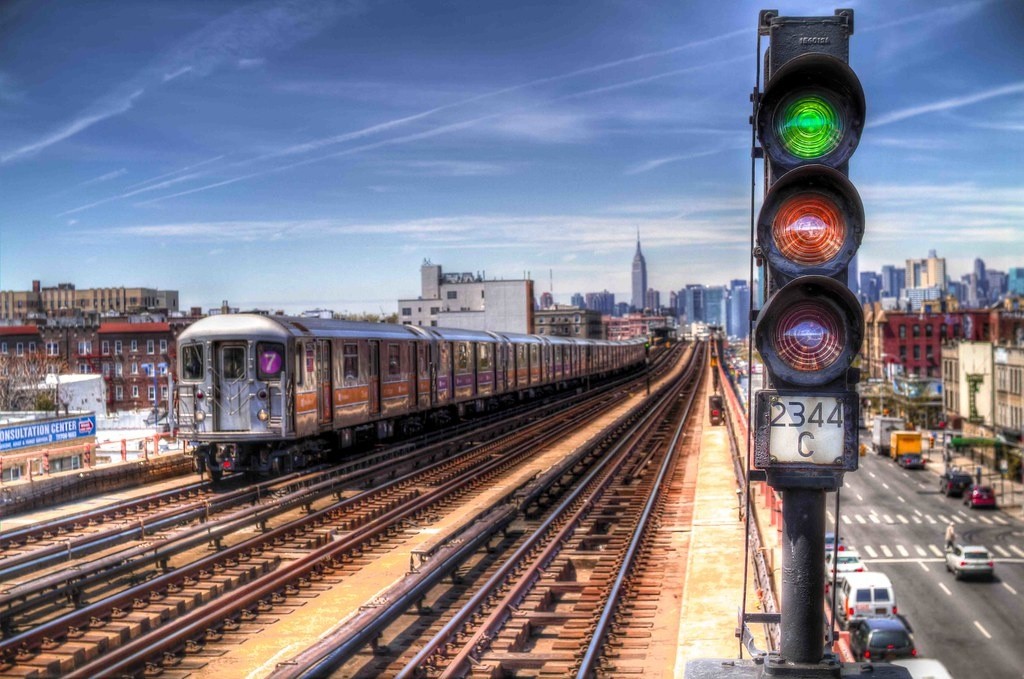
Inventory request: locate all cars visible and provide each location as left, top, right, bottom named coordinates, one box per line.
left=849, top=617, right=918, bottom=664
left=891, top=658, right=954, bottom=679
left=939, top=471, right=973, bottom=497
left=729, top=351, right=764, bottom=381
left=899, top=453, right=927, bottom=469
left=823, top=533, right=870, bottom=596
left=945, top=544, right=995, bottom=579
left=962, top=484, right=997, bottom=508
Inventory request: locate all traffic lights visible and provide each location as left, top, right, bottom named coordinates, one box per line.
left=757, top=51, right=865, bottom=387
left=709, top=397, right=722, bottom=426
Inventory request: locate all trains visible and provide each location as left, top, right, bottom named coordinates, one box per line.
left=174, top=297, right=650, bottom=479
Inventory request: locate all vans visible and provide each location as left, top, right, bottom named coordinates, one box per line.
left=835, top=572, right=901, bottom=631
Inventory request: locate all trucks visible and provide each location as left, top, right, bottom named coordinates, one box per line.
left=889, top=429, right=923, bottom=461
left=871, top=415, right=906, bottom=454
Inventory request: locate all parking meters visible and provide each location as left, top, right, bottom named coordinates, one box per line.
left=868, top=377, right=884, bottom=417
